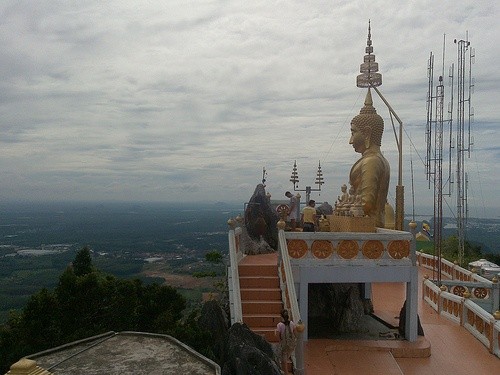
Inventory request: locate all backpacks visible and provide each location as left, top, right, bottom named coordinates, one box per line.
left=280, top=320, right=297, bottom=351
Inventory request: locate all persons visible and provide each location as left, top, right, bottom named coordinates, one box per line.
left=300, top=200, right=317, bottom=226
left=338, top=184, right=348, bottom=204
left=349, top=106, right=391, bottom=227
left=285, top=191, right=297, bottom=232
left=274, top=309, right=297, bottom=369
